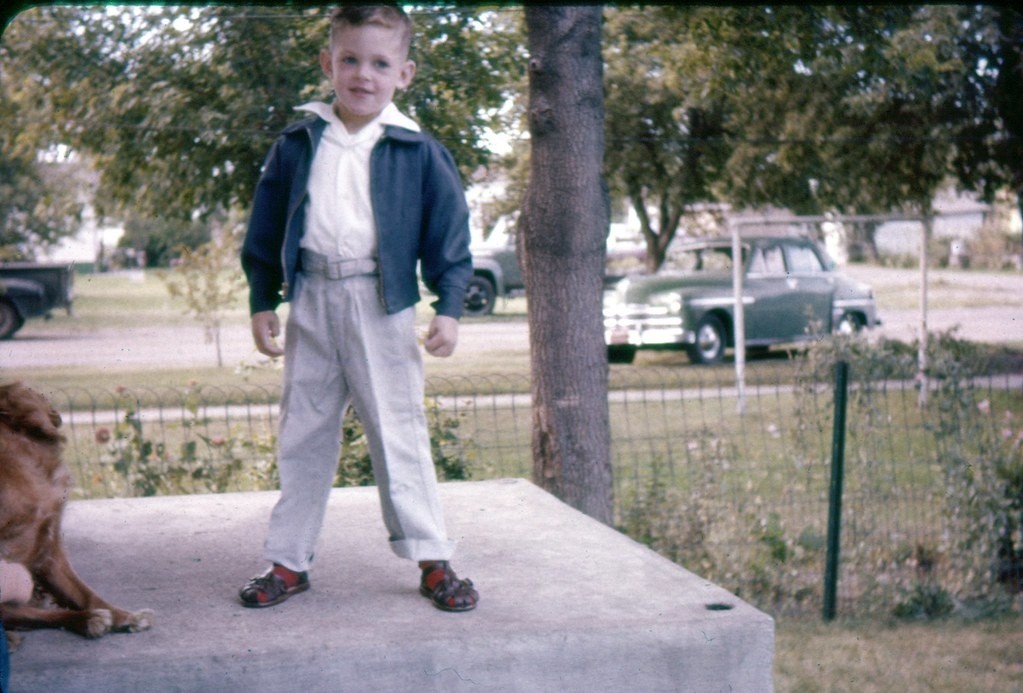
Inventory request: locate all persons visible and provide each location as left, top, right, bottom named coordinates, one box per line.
left=238, top=0, right=481, bottom=614
left=0, top=559, right=36, bottom=693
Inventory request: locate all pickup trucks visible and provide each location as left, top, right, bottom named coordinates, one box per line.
left=0, top=260, right=78, bottom=340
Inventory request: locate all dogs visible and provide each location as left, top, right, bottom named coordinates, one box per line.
left=1, top=382, right=152, bottom=642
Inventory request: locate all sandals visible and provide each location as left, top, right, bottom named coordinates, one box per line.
left=419, top=563, right=479, bottom=610
left=239, top=566, right=309, bottom=606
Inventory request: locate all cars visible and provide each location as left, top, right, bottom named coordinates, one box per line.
left=453, top=211, right=526, bottom=320
left=602, top=233, right=883, bottom=367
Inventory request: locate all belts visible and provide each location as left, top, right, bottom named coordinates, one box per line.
left=296, top=258, right=381, bottom=281
